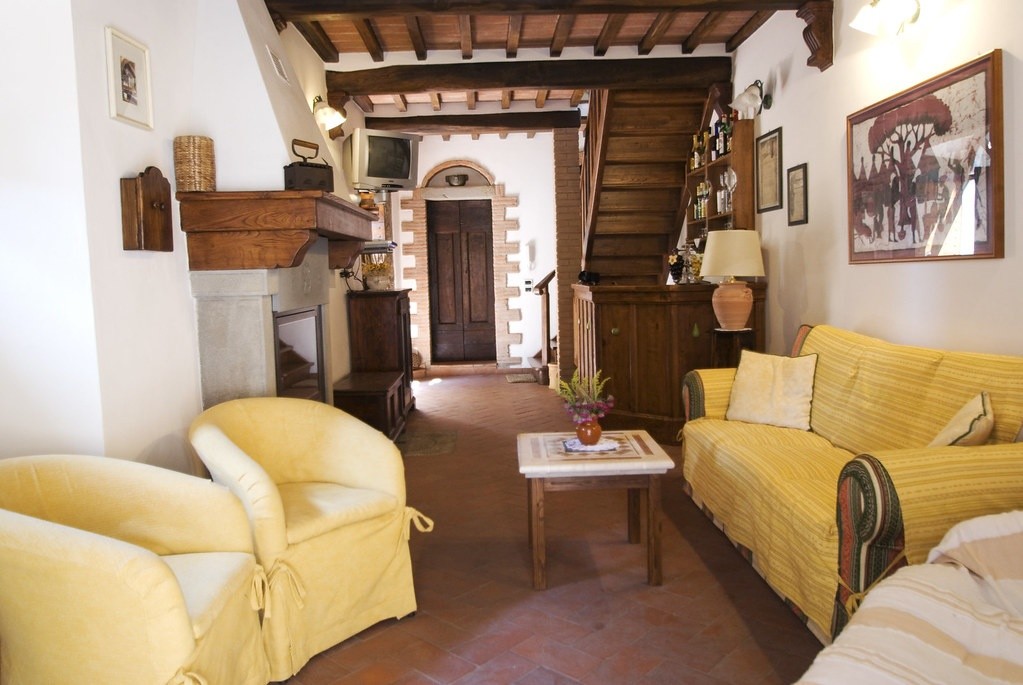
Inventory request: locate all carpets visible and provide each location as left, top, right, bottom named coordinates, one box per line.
left=506, top=373, right=537, bottom=384
left=394, top=431, right=458, bottom=458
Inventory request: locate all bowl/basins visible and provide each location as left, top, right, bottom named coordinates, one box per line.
left=366, top=275, right=389, bottom=291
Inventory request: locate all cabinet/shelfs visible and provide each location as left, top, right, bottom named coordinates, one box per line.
left=685, top=82, right=754, bottom=252
left=120, top=166, right=174, bottom=252
left=333, top=288, right=416, bottom=440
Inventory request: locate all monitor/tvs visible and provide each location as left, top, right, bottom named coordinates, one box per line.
left=352, top=128, right=419, bottom=190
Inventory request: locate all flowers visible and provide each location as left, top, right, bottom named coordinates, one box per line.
left=555, top=369, right=613, bottom=424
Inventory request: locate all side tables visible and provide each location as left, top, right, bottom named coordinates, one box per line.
left=712, top=327, right=755, bottom=368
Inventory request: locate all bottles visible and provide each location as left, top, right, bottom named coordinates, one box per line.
left=716, top=171, right=732, bottom=214
left=693, top=183, right=707, bottom=219
left=710, top=114, right=733, bottom=161
left=689, top=132, right=708, bottom=172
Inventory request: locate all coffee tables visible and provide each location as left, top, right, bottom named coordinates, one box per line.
left=516, top=430, right=676, bottom=591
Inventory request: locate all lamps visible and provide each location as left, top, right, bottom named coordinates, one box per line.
left=728, top=79, right=772, bottom=120
left=312, top=95, right=346, bottom=130
left=699, top=229, right=765, bottom=330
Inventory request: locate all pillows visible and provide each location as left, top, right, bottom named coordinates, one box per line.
left=928, top=391, right=995, bottom=447
left=725, top=348, right=818, bottom=430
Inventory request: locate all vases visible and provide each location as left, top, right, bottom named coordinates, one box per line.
left=576, top=418, right=602, bottom=445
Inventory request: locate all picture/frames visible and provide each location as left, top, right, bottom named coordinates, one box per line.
left=756, top=125, right=783, bottom=214
left=787, top=163, right=808, bottom=226
left=105, top=26, right=154, bottom=131
left=846, top=48, right=1005, bottom=265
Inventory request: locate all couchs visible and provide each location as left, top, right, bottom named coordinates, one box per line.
left=0, top=454, right=272, bottom=685
left=787, top=510, right=1023, bottom=685
left=681, top=324, right=1023, bottom=647
left=189, top=396, right=437, bottom=682
